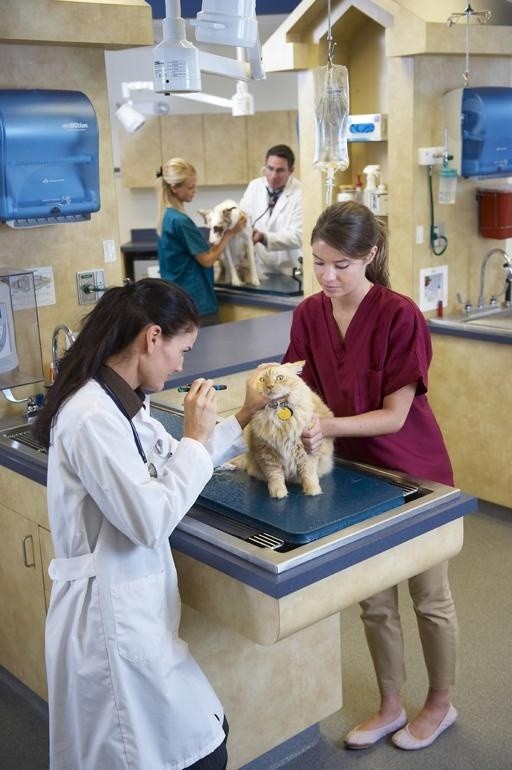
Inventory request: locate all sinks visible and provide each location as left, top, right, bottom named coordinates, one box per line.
left=460, top=309, right=512, bottom=331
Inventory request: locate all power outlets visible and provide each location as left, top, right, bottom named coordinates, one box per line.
left=77, top=269, right=97, bottom=306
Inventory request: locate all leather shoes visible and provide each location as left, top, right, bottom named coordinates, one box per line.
left=343, top=707, right=408, bottom=749
left=391, top=701, right=458, bottom=750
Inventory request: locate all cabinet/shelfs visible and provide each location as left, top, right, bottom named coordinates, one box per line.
left=118, top=110, right=302, bottom=191
left=1, top=465, right=64, bottom=707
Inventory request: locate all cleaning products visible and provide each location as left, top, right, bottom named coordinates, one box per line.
left=362, top=164, right=381, bottom=211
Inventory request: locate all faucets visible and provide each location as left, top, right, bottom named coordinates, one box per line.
left=51, top=322, right=76, bottom=375
left=476, top=248, right=512, bottom=309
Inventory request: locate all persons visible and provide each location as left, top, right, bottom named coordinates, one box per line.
left=238, top=143, right=304, bottom=274
left=282, top=199, right=461, bottom=753
left=154, top=157, right=247, bottom=329
left=31, top=276, right=282, bottom=769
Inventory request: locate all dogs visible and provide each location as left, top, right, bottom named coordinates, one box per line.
left=195, top=198, right=268, bottom=288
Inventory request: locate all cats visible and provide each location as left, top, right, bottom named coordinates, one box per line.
left=224, top=359, right=337, bottom=500
